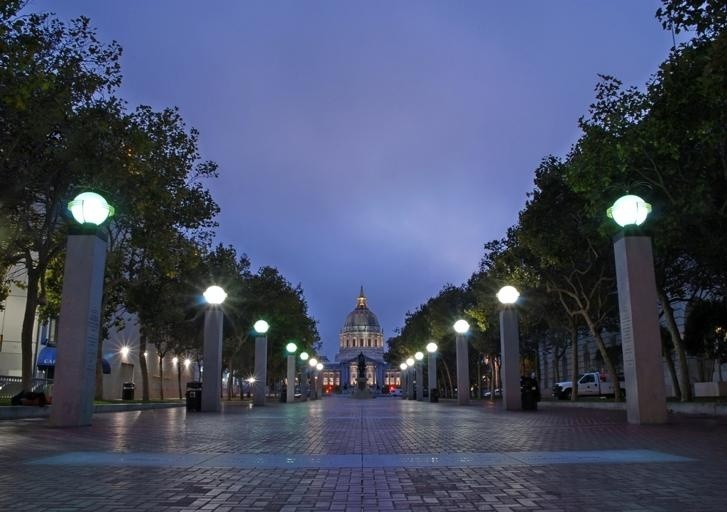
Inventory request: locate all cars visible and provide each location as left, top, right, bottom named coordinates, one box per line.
left=484, top=389, right=503, bottom=398
left=391, top=389, right=402, bottom=396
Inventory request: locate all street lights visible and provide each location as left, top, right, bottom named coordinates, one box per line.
left=426, top=342, right=437, bottom=401
left=286, top=342, right=297, bottom=402
left=253, top=320, right=270, bottom=405
left=497, top=286, right=522, bottom=410
left=454, top=320, right=471, bottom=405
left=400, top=352, right=424, bottom=400
left=300, top=352, right=323, bottom=401
left=48, top=192, right=114, bottom=426
left=200, top=286, right=228, bottom=411
left=606, top=196, right=669, bottom=425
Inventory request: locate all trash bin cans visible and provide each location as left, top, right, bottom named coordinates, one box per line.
left=431, top=389, right=438, bottom=402
left=187, top=382, right=201, bottom=411
left=123, top=382, right=135, bottom=400
left=522, top=387, right=537, bottom=410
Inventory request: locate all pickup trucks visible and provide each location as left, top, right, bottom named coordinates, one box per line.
left=552, top=372, right=626, bottom=400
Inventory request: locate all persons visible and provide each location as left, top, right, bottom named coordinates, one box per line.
left=36, top=366, right=45, bottom=393
left=618, top=367, right=626, bottom=399
left=599, top=367, right=607, bottom=381
